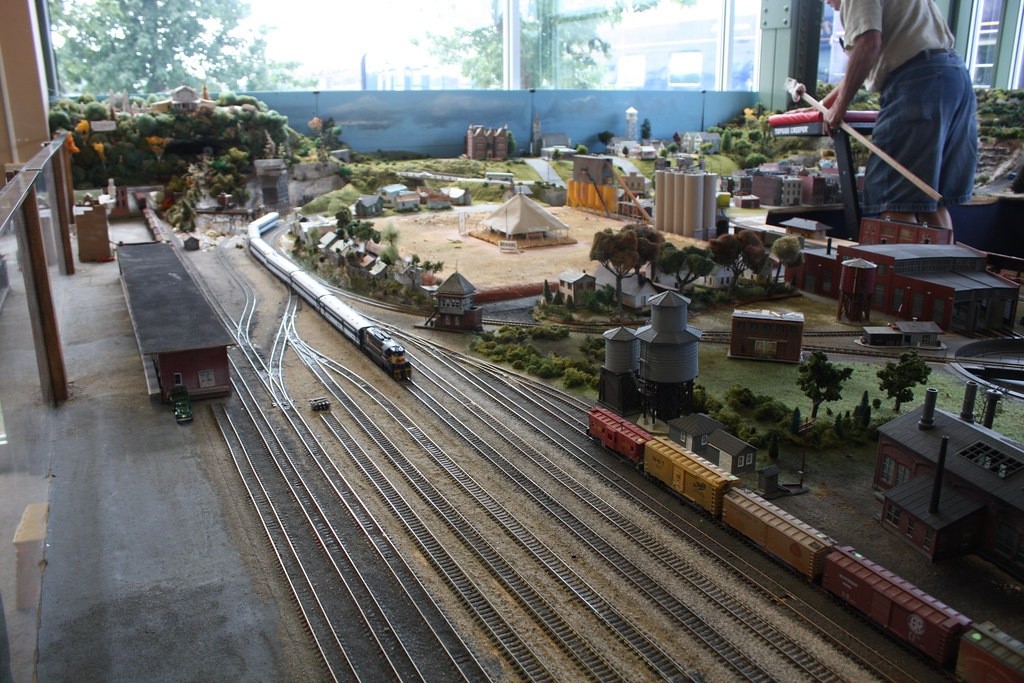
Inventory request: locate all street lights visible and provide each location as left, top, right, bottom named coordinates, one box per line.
left=529, top=90, right=535, bottom=153
left=701, top=90, right=707, bottom=132
left=313, top=91, right=320, bottom=118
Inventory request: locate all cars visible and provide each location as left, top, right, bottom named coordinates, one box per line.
left=174, top=402, right=193, bottom=425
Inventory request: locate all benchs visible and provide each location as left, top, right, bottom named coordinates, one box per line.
left=499, top=240, right=520, bottom=254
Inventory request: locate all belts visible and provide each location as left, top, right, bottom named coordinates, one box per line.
left=887, top=48, right=948, bottom=88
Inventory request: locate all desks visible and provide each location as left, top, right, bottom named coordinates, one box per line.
left=767, top=110, right=879, bottom=241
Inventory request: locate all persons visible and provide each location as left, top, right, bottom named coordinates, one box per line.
left=785, top=0, right=979, bottom=245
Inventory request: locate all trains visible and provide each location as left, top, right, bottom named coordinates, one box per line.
left=247, top=212, right=415, bottom=382
left=584, top=404, right=1024, bottom=683
